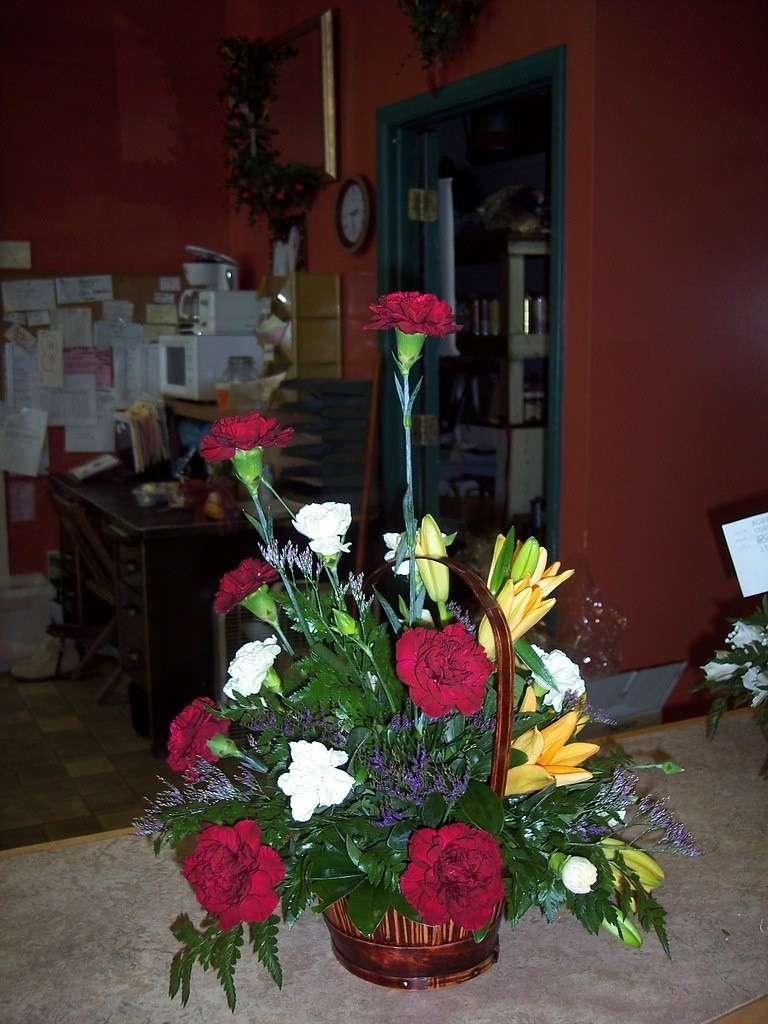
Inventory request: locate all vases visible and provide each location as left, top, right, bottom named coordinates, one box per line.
left=322, top=885, right=502, bottom=991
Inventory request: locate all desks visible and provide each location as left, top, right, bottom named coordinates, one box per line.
left=1, top=706, right=766, bottom=1024
left=51, top=466, right=320, bottom=757
left=165, top=400, right=322, bottom=462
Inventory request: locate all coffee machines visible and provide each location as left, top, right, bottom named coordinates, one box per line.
left=177, top=247, right=241, bottom=335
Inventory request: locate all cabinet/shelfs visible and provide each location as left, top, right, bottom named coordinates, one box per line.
left=439, top=231, right=546, bottom=540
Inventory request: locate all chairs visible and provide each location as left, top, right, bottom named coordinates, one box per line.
left=49, top=492, right=143, bottom=711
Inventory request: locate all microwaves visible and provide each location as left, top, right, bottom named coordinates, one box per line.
left=159, top=335, right=257, bottom=401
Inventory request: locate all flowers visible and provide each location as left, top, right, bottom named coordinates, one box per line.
left=692, top=596, right=768, bottom=746
left=211, top=36, right=323, bottom=227
left=130, top=283, right=708, bottom=1012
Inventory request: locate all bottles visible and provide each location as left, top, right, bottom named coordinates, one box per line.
left=217, top=356, right=263, bottom=414
left=523, top=391, right=545, bottom=421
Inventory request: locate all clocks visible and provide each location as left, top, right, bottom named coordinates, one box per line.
left=332, top=172, right=374, bottom=253
